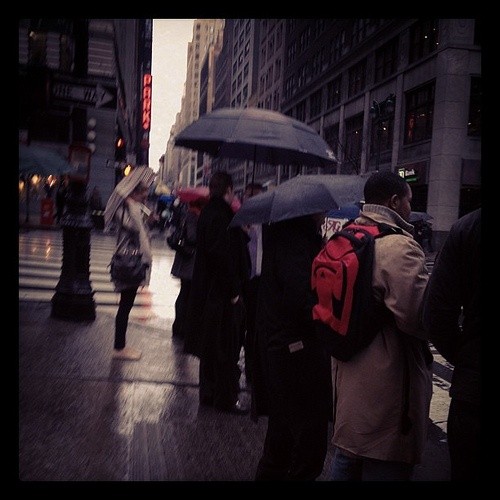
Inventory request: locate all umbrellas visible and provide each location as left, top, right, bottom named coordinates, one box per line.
left=174, top=108, right=337, bottom=198
left=233, top=174, right=368, bottom=226
left=103, top=165, right=149, bottom=232
left=409, top=211, right=433, bottom=222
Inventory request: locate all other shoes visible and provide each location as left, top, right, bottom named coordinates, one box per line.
left=225, top=400, right=246, bottom=413
left=113, top=347, right=143, bottom=360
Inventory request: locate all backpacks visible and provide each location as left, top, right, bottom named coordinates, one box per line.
left=310, top=215, right=408, bottom=363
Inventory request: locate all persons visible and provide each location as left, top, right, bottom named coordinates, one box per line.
left=56, top=169, right=500, bottom=500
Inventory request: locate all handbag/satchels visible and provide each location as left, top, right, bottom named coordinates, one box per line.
left=108, top=231, right=148, bottom=285
